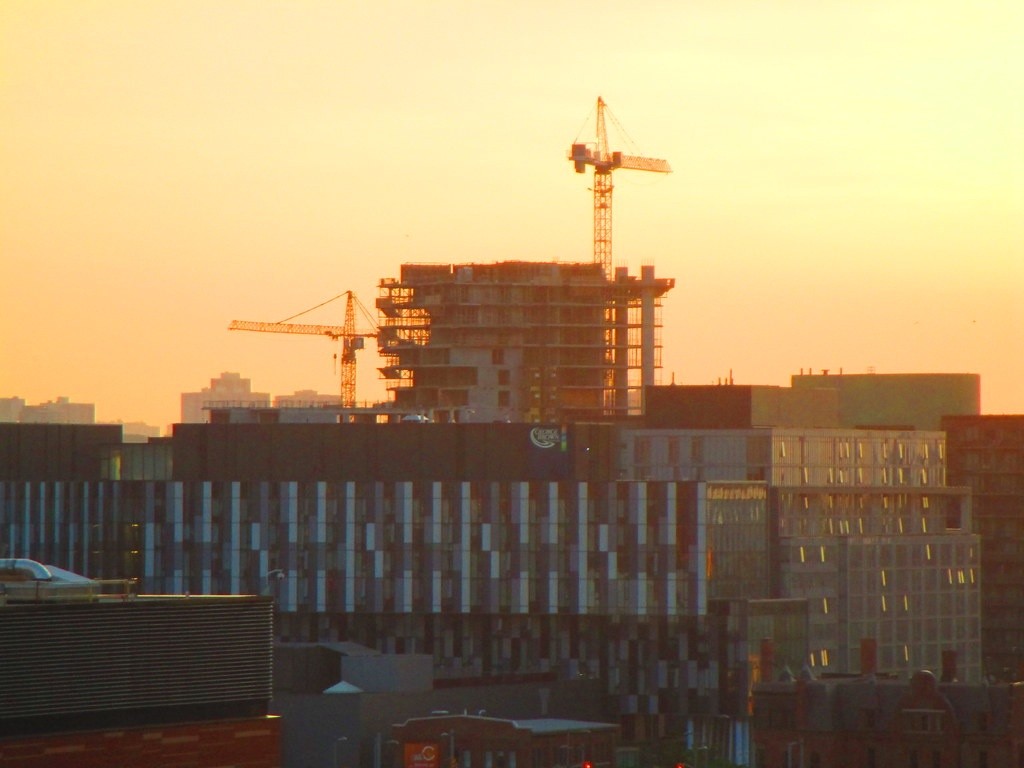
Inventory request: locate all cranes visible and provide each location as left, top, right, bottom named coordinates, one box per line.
left=563, top=89, right=673, bottom=424
left=220, top=287, right=394, bottom=430
